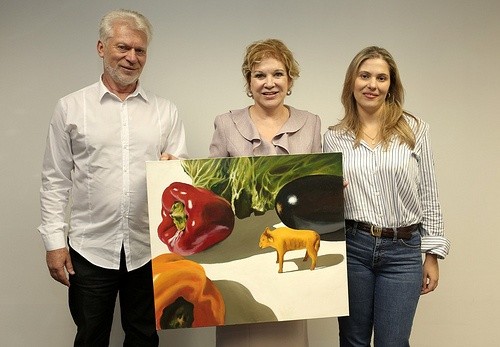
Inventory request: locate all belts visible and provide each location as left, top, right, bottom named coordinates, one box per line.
left=345, top=219, right=418, bottom=239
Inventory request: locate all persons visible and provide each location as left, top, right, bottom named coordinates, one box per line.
left=208, top=38, right=322, bottom=347
left=323, top=47, right=451, bottom=347
left=37, top=9, right=188, bottom=347
